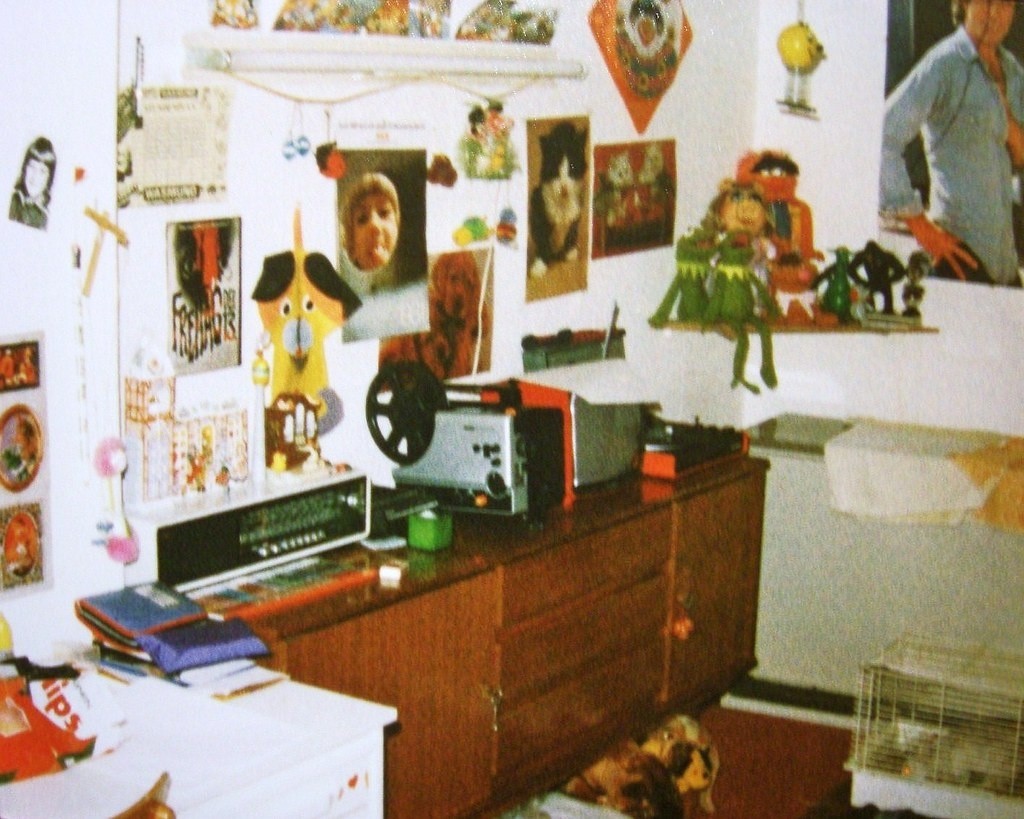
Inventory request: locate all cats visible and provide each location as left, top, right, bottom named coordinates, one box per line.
left=529, top=121, right=590, bottom=279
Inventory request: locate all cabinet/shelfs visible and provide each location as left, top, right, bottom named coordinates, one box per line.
left=241, top=454, right=769, bottom=819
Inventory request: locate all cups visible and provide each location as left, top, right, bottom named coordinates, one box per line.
left=408, top=514, right=455, bottom=551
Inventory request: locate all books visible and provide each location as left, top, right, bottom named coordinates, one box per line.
left=74, top=581, right=281, bottom=706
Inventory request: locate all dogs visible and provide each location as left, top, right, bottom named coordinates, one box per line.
left=379, top=302, right=451, bottom=391
left=632, top=713, right=720, bottom=813
left=432, top=252, right=490, bottom=380
left=901, top=716, right=1024, bottom=793
left=559, top=747, right=683, bottom=819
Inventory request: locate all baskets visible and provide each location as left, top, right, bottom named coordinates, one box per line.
left=851, top=631, right=1023, bottom=801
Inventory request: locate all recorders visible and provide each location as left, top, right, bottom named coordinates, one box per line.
left=126, top=463, right=375, bottom=596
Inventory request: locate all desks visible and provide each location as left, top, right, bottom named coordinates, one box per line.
left=0, top=657, right=398, bottom=819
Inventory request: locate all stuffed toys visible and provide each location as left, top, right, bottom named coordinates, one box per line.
left=699, top=229, right=781, bottom=396
left=648, top=229, right=731, bottom=347
left=641, top=714, right=724, bottom=813
left=732, top=149, right=827, bottom=302
left=564, top=737, right=687, bottom=819
left=698, top=176, right=783, bottom=318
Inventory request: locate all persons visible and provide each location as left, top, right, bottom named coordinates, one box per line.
left=877, top=0, right=1023, bottom=294
left=338, top=170, right=402, bottom=280
